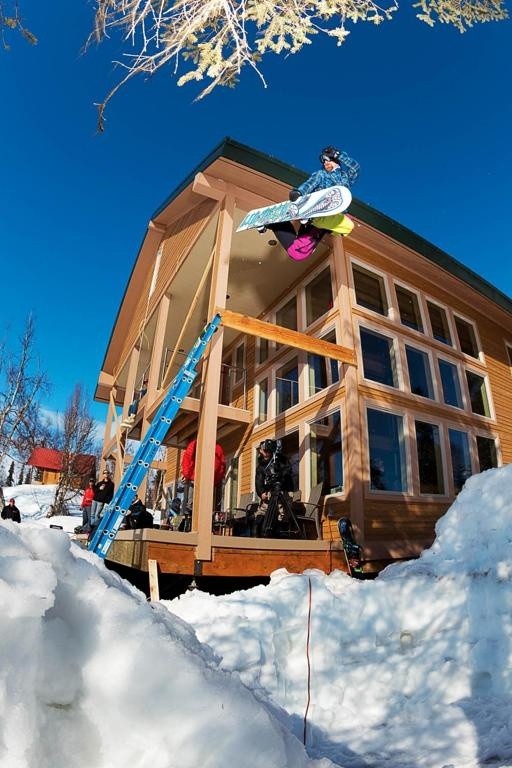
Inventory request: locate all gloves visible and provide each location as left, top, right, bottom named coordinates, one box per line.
left=289, top=190, right=299, bottom=201
left=324, top=145, right=338, bottom=158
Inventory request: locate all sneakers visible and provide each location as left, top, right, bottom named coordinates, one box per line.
left=257, top=226, right=267, bottom=233
left=300, top=218, right=310, bottom=227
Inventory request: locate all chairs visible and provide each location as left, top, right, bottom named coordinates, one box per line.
left=212, top=481, right=325, bottom=539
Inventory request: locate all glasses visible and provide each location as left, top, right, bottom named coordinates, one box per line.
left=319, top=154, right=331, bottom=163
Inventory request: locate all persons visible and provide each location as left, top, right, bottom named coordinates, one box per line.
left=254, top=441, right=292, bottom=540
left=91, top=469, right=115, bottom=524
left=125, top=493, right=144, bottom=530
left=1, top=498, right=21, bottom=523
left=80, top=478, right=95, bottom=526
left=168, top=497, right=181, bottom=517
left=120, top=378, right=148, bottom=429
left=181, top=438, right=226, bottom=517
left=265, top=145, right=361, bottom=263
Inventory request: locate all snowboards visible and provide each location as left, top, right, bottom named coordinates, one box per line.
left=236, top=186, right=352, bottom=232
left=338, top=518, right=363, bottom=581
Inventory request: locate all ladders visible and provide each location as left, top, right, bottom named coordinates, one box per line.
left=87, top=314, right=222, bottom=563
